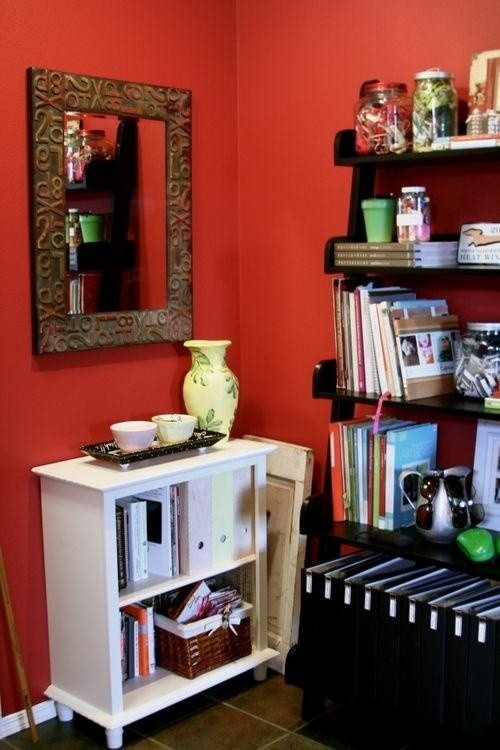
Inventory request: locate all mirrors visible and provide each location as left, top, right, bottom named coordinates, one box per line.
left=28, top=63, right=194, bottom=354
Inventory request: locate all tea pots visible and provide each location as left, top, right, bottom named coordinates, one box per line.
left=399, top=463, right=473, bottom=544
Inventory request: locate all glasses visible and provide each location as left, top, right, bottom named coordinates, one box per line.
left=462, top=473, right=485, bottom=526
left=412, top=473, right=441, bottom=530
left=444, top=472, right=469, bottom=530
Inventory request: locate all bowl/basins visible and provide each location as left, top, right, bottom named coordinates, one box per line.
left=109, top=414, right=197, bottom=452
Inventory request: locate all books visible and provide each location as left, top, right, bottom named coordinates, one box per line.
left=334, top=258, right=459, bottom=269
left=335, top=242, right=459, bottom=251
left=326, top=277, right=463, bottom=532
left=117, top=486, right=182, bottom=682
left=334, top=250, right=458, bottom=260
left=68, top=272, right=133, bottom=314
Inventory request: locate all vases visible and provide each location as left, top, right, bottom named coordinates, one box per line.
left=181, top=339, right=240, bottom=444
left=78, top=214, right=105, bottom=244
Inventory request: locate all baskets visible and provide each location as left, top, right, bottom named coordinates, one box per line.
left=152, top=616, right=252, bottom=680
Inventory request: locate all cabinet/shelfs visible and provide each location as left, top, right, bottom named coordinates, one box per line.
left=64, top=121, right=139, bottom=312
left=30, top=436, right=278, bottom=749
left=284, top=120, right=500, bottom=718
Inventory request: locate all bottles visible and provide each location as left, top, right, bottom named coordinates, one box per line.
left=454, top=320, right=500, bottom=402
left=73, top=127, right=114, bottom=184
left=395, top=186, right=431, bottom=242
left=353, top=67, right=499, bottom=156
